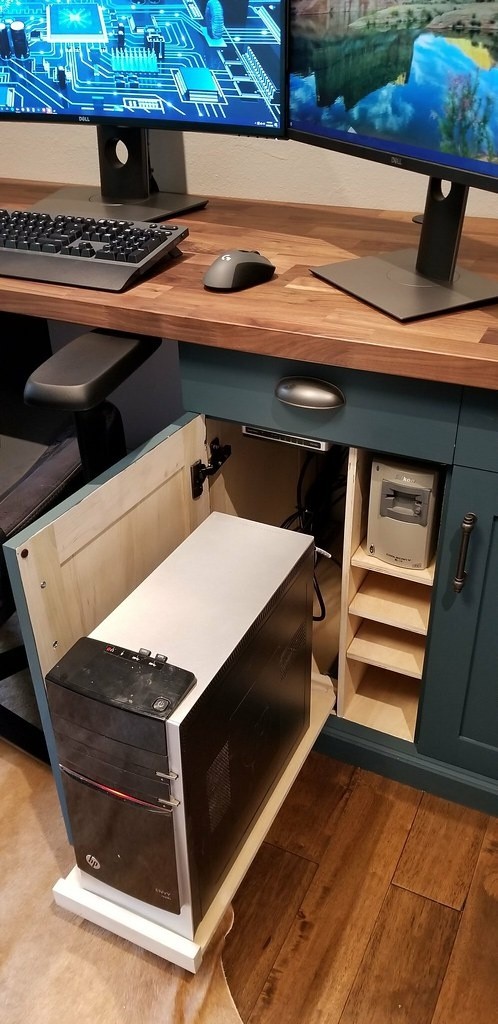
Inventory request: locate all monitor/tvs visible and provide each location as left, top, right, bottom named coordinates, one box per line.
left=0, top=0, right=292, bottom=227
left=286, top=0, right=498, bottom=324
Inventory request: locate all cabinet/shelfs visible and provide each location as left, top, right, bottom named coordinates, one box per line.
left=0, top=340, right=498, bottom=844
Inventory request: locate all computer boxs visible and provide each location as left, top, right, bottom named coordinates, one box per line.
left=43, top=511, right=313, bottom=941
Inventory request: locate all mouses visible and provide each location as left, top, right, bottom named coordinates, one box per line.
left=204, top=249, right=276, bottom=290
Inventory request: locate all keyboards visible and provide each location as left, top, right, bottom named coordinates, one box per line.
left=0, top=207, right=189, bottom=293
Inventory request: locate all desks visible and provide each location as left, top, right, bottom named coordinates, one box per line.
left=0, top=174, right=498, bottom=394
